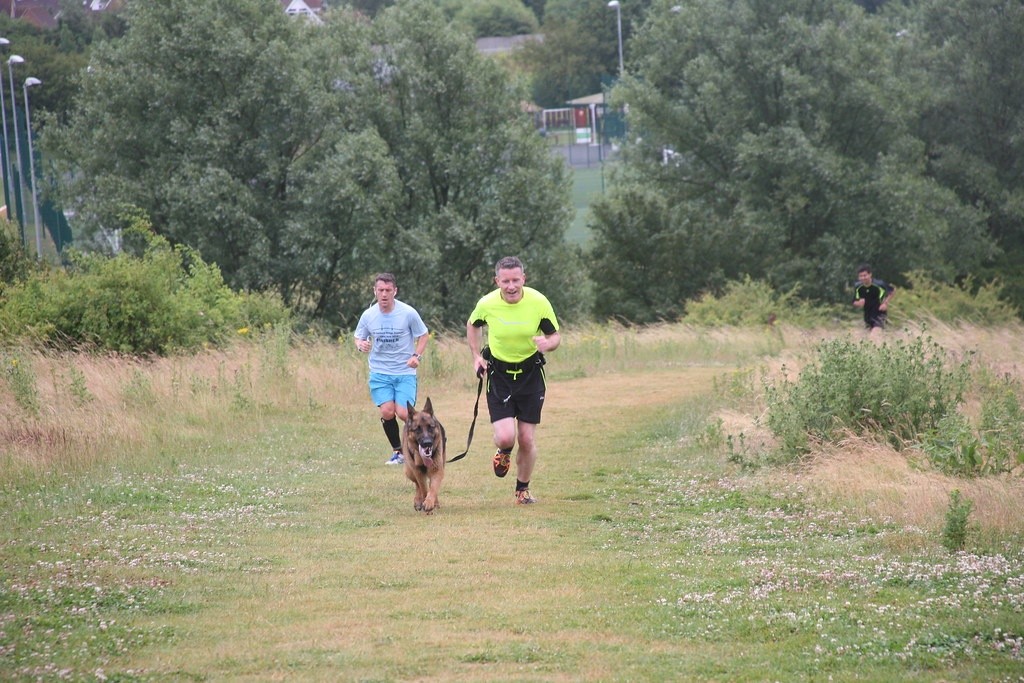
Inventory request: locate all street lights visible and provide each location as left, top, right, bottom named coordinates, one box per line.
left=23, top=77, right=41, bottom=263
left=607, top=0, right=626, bottom=76
left=7, top=54, right=25, bottom=246
left=0, top=33, right=11, bottom=201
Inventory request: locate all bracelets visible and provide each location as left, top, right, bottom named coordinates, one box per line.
left=358, top=348, right=361, bottom=351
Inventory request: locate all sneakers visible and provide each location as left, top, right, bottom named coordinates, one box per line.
left=515, top=489, right=536, bottom=504
left=493, top=447, right=513, bottom=477
left=385, top=451, right=403, bottom=465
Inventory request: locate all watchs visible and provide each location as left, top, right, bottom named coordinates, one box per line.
left=413, top=354, right=422, bottom=360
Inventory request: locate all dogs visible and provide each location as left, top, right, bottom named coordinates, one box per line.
left=401, top=397, right=447, bottom=515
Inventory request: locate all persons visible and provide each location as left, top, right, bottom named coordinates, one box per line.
left=853, top=266, right=896, bottom=339
left=467, top=256, right=561, bottom=504
left=355, top=272, right=429, bottom=464
